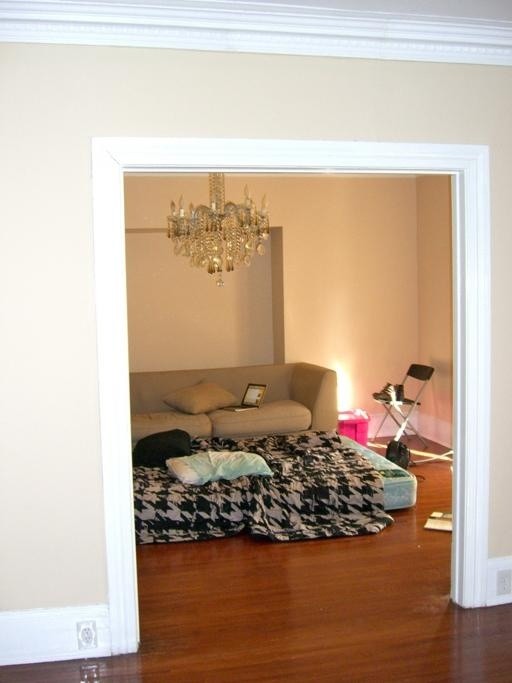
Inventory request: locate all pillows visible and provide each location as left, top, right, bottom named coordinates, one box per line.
left=167, top=451, right=273, bottom=485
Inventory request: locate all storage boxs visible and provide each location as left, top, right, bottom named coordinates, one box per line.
left=338, top=410, right=369, bottom=447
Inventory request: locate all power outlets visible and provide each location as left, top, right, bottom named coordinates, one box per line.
left=77, top=620, right=97, bottom=651
left=497, top=569, right=512, bottom=594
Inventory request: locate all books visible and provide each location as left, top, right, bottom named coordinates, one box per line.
left=423, top=510, right=453, bottom=532
left=222, top=405, right=258, bottom=412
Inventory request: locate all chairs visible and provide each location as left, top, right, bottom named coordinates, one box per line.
left=371, top=365, right=435, bottom=448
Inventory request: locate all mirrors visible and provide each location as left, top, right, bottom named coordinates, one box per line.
left=163, top=381, right=236, bottom=414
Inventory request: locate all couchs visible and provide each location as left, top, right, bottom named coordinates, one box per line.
left=131, top=362, right=338, bottom=447
left=133, top=432, right=417, bottom=542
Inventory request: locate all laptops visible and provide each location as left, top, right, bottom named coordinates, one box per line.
left=224, top=383, right=268, bottom=412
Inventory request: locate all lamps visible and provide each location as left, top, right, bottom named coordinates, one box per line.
left=165, top=172, right=270, bottom=286
